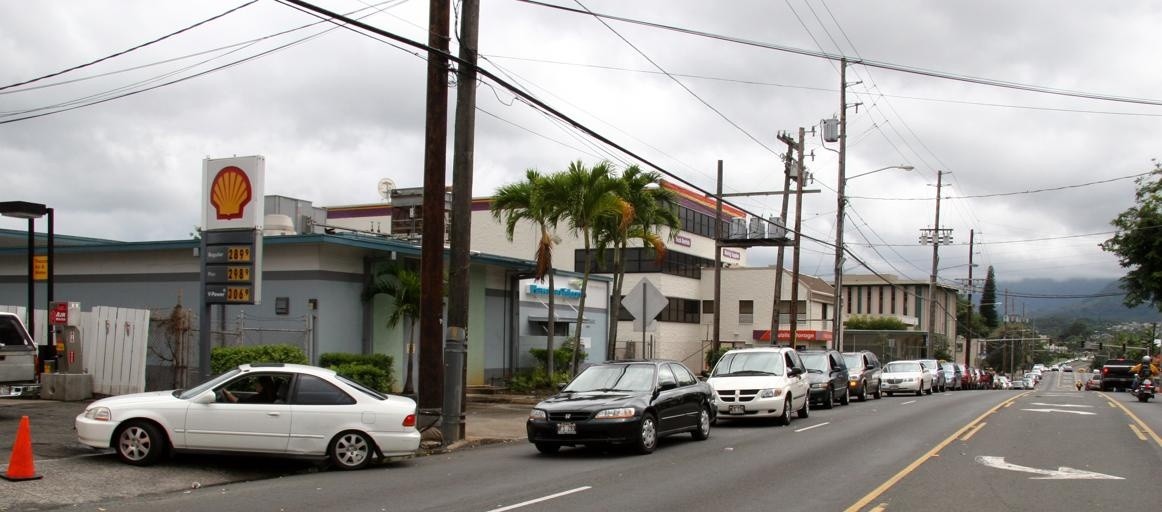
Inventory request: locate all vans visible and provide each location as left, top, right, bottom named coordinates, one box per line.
left=840, top=351, right=882, bottom=402
left=0, top=312, right=43, bottom=399
left=797, top=350, right=851, bottom=409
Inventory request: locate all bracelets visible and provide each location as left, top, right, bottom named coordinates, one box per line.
left=227, top=391, right=231, bottom=396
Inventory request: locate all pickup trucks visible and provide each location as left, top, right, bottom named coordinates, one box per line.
left=1101, top=358, right=1138, bottom=392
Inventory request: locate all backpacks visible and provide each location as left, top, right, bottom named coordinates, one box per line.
left=1141, top=363, right=1151, bottom=377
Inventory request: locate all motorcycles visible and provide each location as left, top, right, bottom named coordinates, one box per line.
left=1130, top=373, right=1159, bottom=403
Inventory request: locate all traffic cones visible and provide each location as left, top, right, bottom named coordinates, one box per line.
left=1, top=415, right=44, bottom=482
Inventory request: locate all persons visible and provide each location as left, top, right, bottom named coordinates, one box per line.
left=1076, top=380, right=1083, bottom=392
left=986, top=368, right=996, bottom=384
left=1128, top=356, right=1159, bottom=392
left=222, top=377, right=275, bottom=404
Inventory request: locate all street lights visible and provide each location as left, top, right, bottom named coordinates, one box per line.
left=835, top=165, right=915, bottom=350
left=0, top=199, right=54, bottom=348
left=966, top=302, right=1003, bottom=366
left=927, top=264, right=979, bottom=359
left=1003, top=328, right=1031, bottom=375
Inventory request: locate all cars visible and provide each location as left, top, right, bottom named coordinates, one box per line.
left=75, top=363, right=421, bottom=470
left=882, top=354, right=1088, bottom=397
left=1085, top=374, right=1101, bottom=391
left=706, top=346, right=811, bottom=426
left=527, top=359, right=718, bottom=455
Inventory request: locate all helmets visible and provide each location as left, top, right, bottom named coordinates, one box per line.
left=1142, top=356, right=1150, bottom=363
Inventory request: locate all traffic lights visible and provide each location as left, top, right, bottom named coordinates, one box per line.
left=1049, top=338, right=1053, bottom=346
left=1123, top=343, right=1126, bottom=352
left=1099, top=342, right=1103, bottom=350
left=1081, top=340, right=1085, bottom=348
